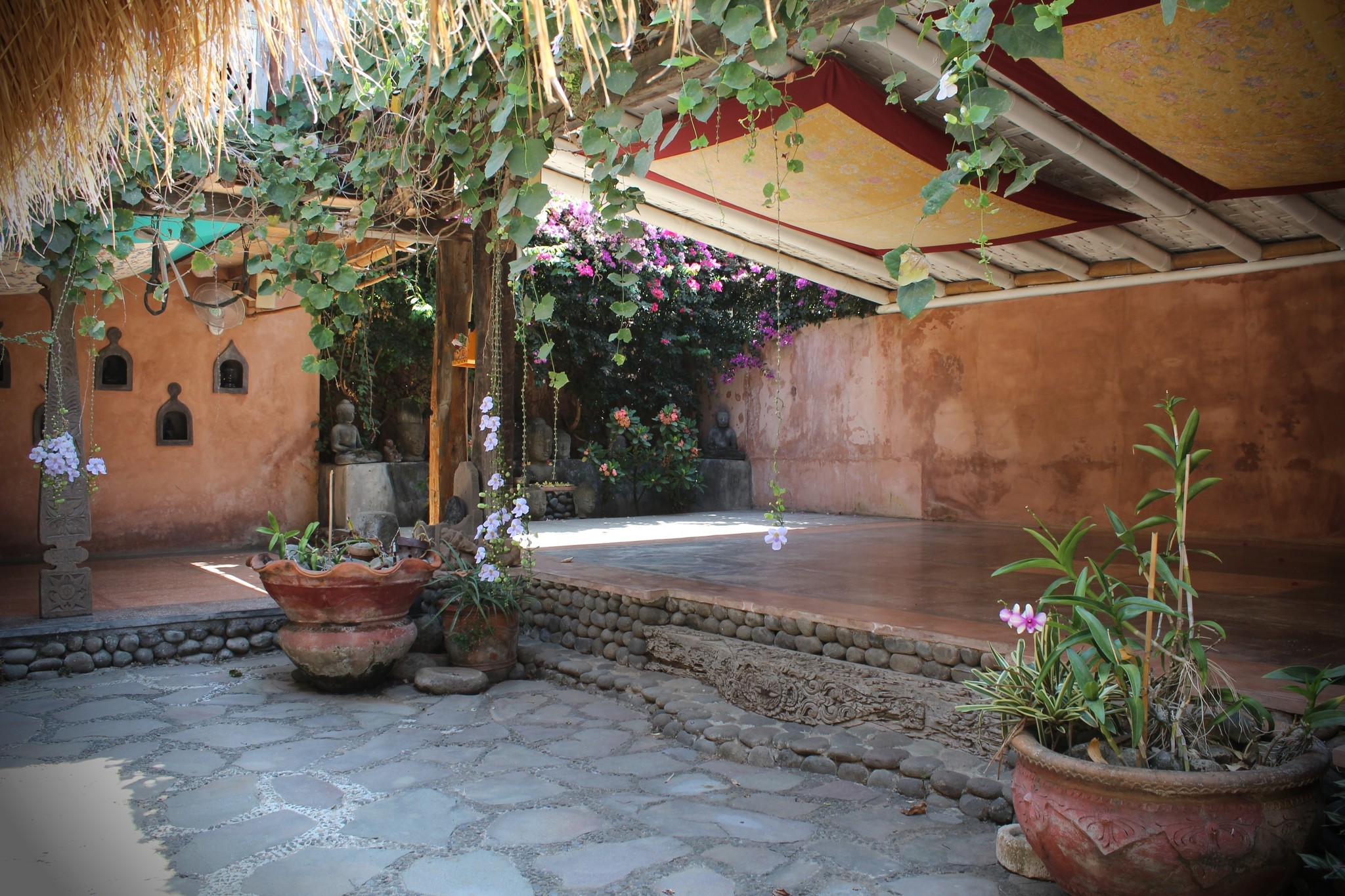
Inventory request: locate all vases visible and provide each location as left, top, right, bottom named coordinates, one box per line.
left=1002, top=724, right=1333, bottom=895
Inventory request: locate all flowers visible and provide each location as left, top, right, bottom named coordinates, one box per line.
left=968, top=465, right=1344, bottom=764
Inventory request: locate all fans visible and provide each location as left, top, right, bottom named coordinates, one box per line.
left=193, top=281, right=245, bottom=337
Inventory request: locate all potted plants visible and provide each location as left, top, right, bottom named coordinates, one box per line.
left=239, top=515, right=445, bottom=679
left=420, top=536, right=524, bottom=680
